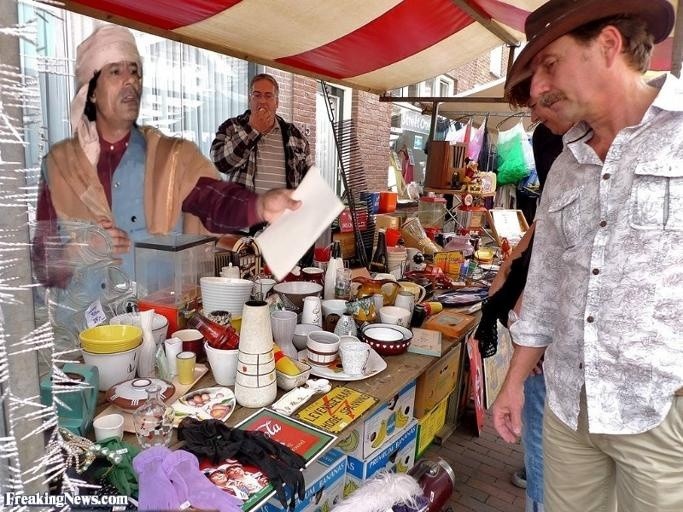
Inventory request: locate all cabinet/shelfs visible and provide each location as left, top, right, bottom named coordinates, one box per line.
left=428, top=139, right=467, bottom=189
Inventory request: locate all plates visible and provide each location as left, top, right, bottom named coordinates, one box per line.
left=297, top=342, right=387, bottom=382
left=169, top=386, right=236, bottom=428
left=433, top=289, right=489, bottom=306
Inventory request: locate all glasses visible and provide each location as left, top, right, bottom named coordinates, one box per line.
left=248, top=91, right=277, bottom=101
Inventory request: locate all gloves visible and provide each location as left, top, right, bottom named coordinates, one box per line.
left=97, top=419, right=306, bottom=512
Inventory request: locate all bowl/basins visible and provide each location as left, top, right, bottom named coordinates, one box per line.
left=273, top=277, right=413, bottom=355
left=79, top=276, right=253, bottom=414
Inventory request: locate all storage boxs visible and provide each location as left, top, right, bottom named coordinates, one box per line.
left=332, top=190, right=402, bottom=259
left=260, top=342, right=466, bottom=511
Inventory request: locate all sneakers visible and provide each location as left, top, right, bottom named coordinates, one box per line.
left=511, top=467, right=527, bottom=489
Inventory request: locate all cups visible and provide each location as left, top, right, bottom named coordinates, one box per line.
left=93, top=413, right=124, bottom=443
left=255, top=267, right=372, bottom=374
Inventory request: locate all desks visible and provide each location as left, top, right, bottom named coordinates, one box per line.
left=74, top=310, right=487, bottom=512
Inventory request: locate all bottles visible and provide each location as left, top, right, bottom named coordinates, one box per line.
left=132, top=385, right=174, bottom=449
left=234, top=302, right=277, bottom=408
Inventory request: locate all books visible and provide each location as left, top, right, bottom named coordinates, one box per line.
left=407, top=327, right=442, bottom=357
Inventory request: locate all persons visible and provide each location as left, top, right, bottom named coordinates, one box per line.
left=490, top=0, right=683, bottom=512
left=208, top=74, right=316, bottom=271
left=218, top=463, right=270, bottom=495
left=201, top=468, right=250, bottom=504
left=30, top=24, right=304, bottom=370
left=475, top=72, right=564, bottom=512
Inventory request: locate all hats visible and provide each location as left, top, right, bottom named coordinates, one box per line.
left=504, top=1, right=674, bottom=88
